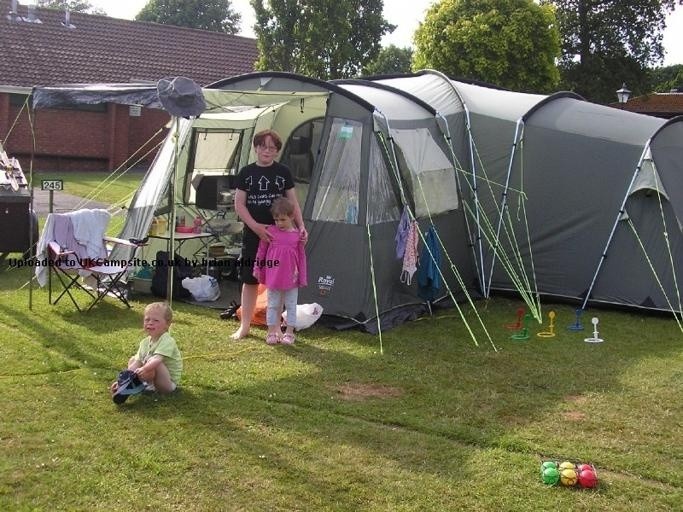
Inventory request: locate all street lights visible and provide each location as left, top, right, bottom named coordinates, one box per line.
left=615, top=81, right=632, bottom=110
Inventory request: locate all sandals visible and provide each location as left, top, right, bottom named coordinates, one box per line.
left=266, top=331, right=295, bottom=345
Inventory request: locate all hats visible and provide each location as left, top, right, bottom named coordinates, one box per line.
left=157, top=76, right=206, bottom=116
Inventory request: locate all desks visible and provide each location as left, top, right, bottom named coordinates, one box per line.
left=146, top=226, right=213, bottom=275
left=0, top=158, right=31, bottom=252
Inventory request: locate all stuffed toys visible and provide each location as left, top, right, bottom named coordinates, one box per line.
left=537, top=460, right=602, bottom=486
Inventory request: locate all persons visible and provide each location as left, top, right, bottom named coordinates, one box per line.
left=112, top=303, right=183, bottom=393
left=253, top=197, right=308, bottom=344
left=229, top=129, right=308, bottom=339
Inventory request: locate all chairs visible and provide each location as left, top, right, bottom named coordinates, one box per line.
left=46, top=209, right=144, bottom=311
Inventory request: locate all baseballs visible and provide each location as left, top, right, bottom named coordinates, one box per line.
left=541, top=460, right=596, bottom=489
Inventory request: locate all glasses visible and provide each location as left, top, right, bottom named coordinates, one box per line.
left=261, top=144, right=277, bottom=152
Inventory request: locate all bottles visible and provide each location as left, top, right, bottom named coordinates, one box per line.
left=175, top=206, right=186, bottom=227
left=150, top=216, right=166, bottom=236
left=193, top=215, right=202, bottom=235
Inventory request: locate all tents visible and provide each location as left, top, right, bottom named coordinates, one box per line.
left=98, top=69, right=682, bottom=333
